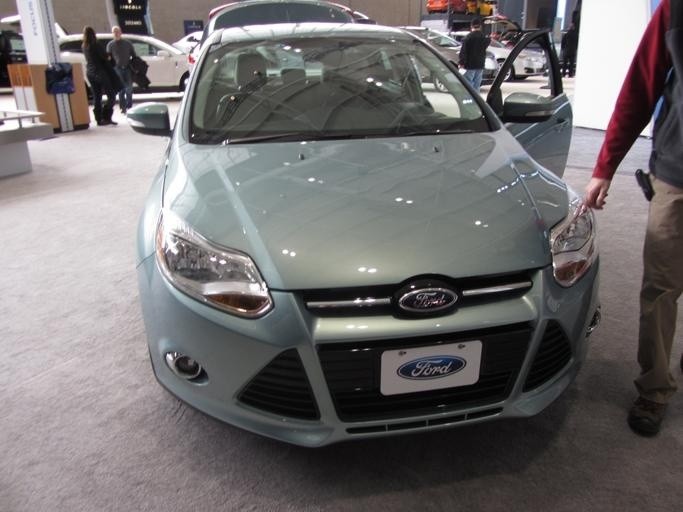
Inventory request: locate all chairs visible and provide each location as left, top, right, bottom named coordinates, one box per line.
left=215, top=51, right=279, bottom=122
left=282, top=68, right=311, bottom=110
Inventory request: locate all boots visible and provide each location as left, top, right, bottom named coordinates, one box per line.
left=94, top=106, right=117, bottom=124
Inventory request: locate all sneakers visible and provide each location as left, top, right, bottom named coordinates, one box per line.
left=628, top=397, right=666, bottom=434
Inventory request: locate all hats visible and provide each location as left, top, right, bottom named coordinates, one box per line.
left=472, top=19, right=480, bottom=25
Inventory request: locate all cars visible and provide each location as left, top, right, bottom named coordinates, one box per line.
left=446, top=32, right=548, bottom=82
left=425, top=0, right=469, bottom=16
left=464, top=0, right=493, bottom=17
left=0, top=31, right=28, bottom=89
left=399, top=27, right=498, bottom=93
left=169, top=30, right=203, bottom=52
left=124, top=0, right=602, bottom=452
left=186, top=44, right=319, bottom=78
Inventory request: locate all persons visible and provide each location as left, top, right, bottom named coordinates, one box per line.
left=82, top=25, right=118, bottom=128
left=584, top=0, right=682, bottom=436
left=106, top=25, right=140, bottom=115
left=458, top=19, right=493, bottom=94
left=0, top=34, right=13, bottom=87
left=561, top=20, right=579, bottom=77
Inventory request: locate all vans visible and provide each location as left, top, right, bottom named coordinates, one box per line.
left=0, top=13, right=196, bottom=101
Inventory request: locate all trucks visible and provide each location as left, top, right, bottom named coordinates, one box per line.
left=419, top=15, right=522, bottom=36
